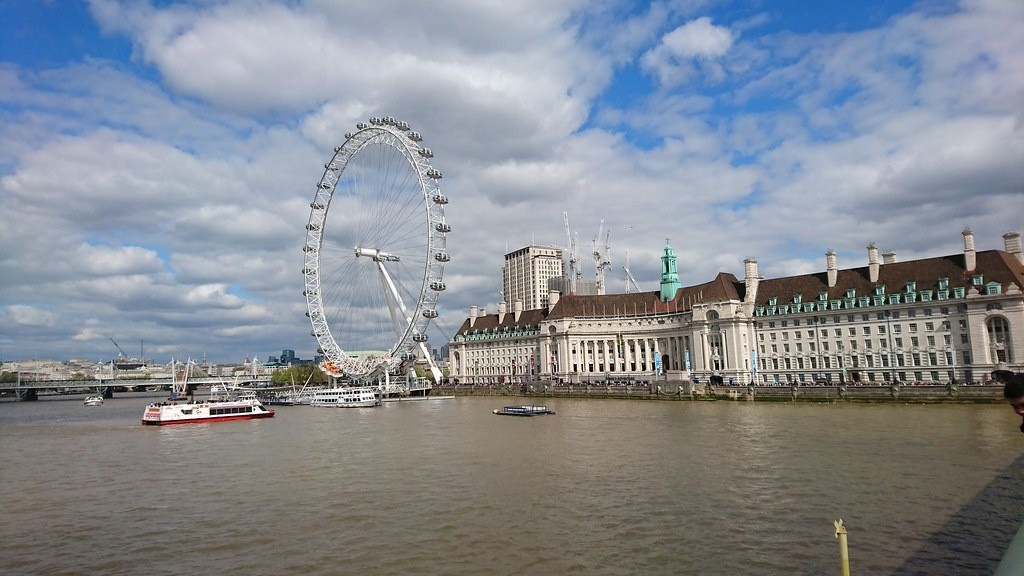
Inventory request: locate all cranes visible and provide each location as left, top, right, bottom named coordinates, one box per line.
left=551, top=211, right=642, bottom=295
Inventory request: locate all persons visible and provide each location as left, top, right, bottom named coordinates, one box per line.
left=899, top=379, right=952, bottom=386
left=719, top=382, right=746, bottom=386
left=959, top=379, right=1005, bottom=384
left=847, top=380, right=893, bottom=386
left=556, top=377, right=651, bottom=386
left=1004, top=373, right=1024, bottom=433
left=760, top=382, right=792, bottom=386
left=151, top=401, right=177, bottom=407
left=802, top=381, right=840, bottom=386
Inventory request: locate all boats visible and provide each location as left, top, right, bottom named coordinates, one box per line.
left=84, top=396, right=103, bottom=406
left=140, top=394, right=275, bottom=425
left=308, top=388, right=379, bottom=408
